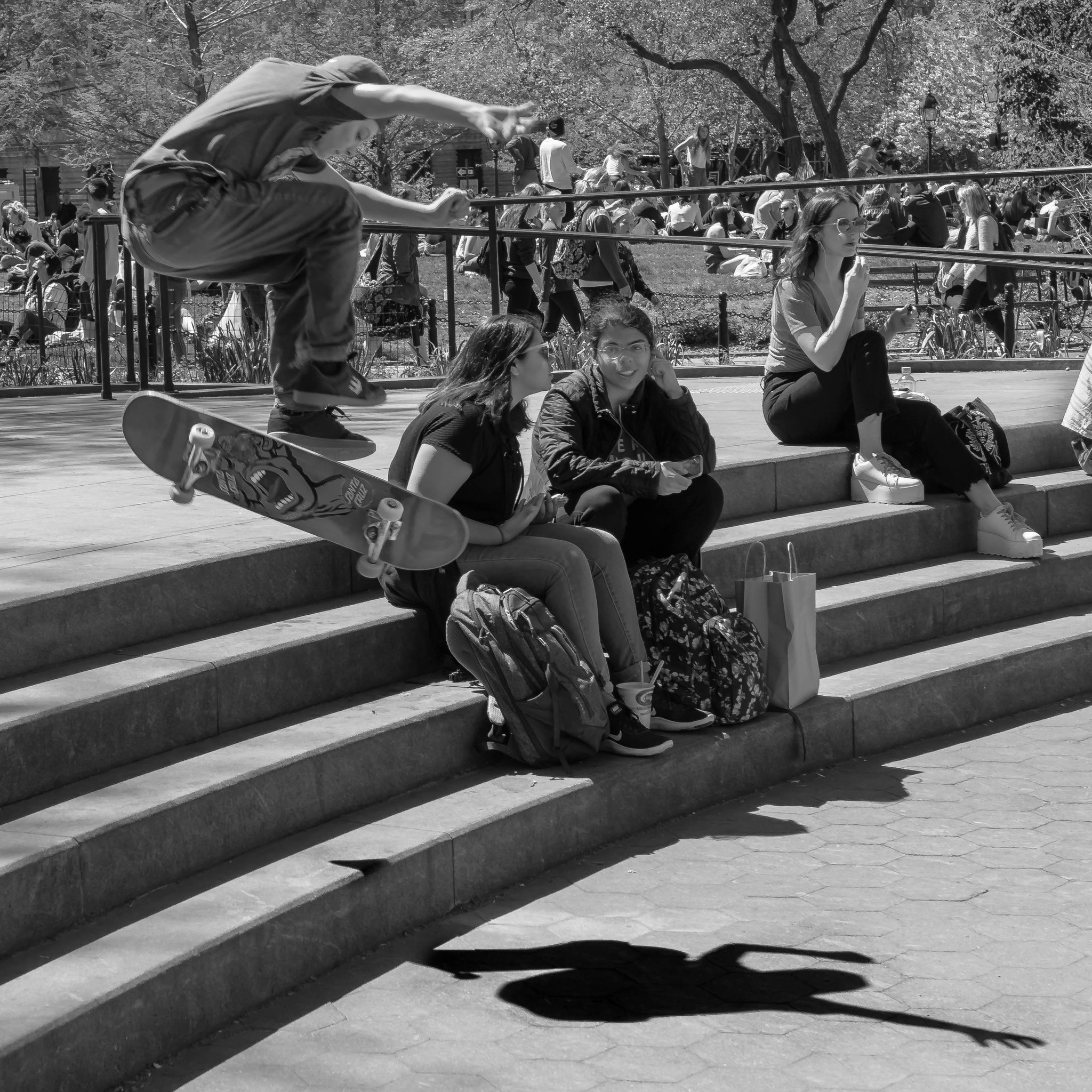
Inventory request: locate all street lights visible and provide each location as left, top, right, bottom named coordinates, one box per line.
left=920, top=84, right=942, bottom=174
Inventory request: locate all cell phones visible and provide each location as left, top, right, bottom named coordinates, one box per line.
left=678, top=454, right=703, bottom=479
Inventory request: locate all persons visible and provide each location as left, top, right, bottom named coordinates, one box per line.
left=762, top=190, right=1043, bottom=558
left=0, top=178, right=266, bottom=363
left=379, top=314, right=716, bottom=756
left=519, top=305, right=722, bottom=568
left=362, top=117, right=1092, bottom=361
left=123, top=54, right=533, bottom=460
left=1061, top=345, right=1092, bottom=474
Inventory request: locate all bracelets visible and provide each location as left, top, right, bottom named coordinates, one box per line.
left=947, top=272, right=955, bottom=278
left=495, top=525, right=504, bottom=544
left=735, top=248, right=739, bottom=254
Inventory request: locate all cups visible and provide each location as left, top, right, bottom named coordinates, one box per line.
left=617, top=682, right=656, bottom=727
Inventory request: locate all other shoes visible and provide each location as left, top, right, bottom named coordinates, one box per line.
left=1, top=338, right=15, bottom=353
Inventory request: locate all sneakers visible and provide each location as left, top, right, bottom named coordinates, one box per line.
left=648, top=685, right=714, bottom=730
left=293, top=357, right=386, bottom=407
left=266, top=404, right=376, bottom=462
left=599, top=697, right=673, bottom=759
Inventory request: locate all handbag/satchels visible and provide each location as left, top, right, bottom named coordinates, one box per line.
left=629, top=215, right=658, bottom=245
left=43, top=279, right=80, bottom=333
left=478, top=239, right=503, bottom=280
left=977, top=213, right=1018, bottom=299
left=733, top=540, right=820, bottom=709
left=348, top=270, right=397, bottom=324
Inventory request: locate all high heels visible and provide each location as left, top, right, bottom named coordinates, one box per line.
left=976, top=500, right=1043, bottom=559
left=850, top=451, right=925, bottom=505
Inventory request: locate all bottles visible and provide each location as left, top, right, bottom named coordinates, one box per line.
left=899, top=366, right=917, bottom=392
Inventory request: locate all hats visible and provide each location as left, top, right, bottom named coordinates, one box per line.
left=775, top=172, right=794, bottom=181
left=547, top=115, right=566, bottom=137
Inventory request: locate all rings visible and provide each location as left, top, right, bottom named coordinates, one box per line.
left=623, top=294, right=626, bottom=295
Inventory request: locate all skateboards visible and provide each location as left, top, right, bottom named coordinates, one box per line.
left=120, top=390, right=469, bottom=578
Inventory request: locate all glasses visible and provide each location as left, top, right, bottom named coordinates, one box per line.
left=793, top=189, right=799, bottom=194
left=520, top=341, right=551, bottom=359
left=820, top=217, right=869, bottom=234
left=38, top=255, right=47, bottom=265
left=778, top=206, right=790, bottom=212
left=728, top=211, right=735, bottom=215
left=597, top=341, right=652, bottom=360
left=68, top=260, right=75, bottom=265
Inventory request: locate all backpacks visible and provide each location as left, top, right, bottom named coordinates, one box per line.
left=942, top=398, right=1012, bottom=489
left=444, top=568, right=605, bottom=772
left=549, top=203, right=604, bottom=280
left=629, top=547, right=770, bottom=726
